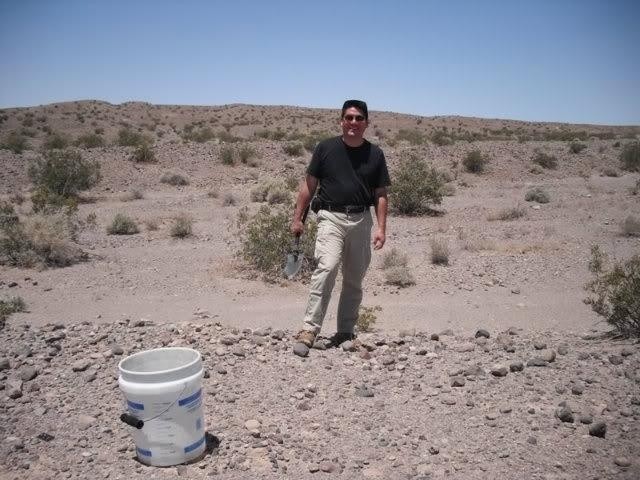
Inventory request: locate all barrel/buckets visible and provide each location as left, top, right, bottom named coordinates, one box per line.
left=119, top=348, right=207, bottom=467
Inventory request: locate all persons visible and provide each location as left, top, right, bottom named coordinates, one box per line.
left=289, top=100, right=392, bottom=358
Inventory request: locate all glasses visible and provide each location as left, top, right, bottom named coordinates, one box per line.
left=343, top=115, right=366, bottom=122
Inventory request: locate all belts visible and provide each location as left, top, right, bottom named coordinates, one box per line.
left=322, top=203, right=370, bottom=213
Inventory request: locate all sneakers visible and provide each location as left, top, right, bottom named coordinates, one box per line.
left=298, top=330, right=316, bottom=348
left=336, top=333, right=354, bottom=343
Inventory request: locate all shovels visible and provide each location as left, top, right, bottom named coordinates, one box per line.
left=282, top=203, right=310, bottom=281
